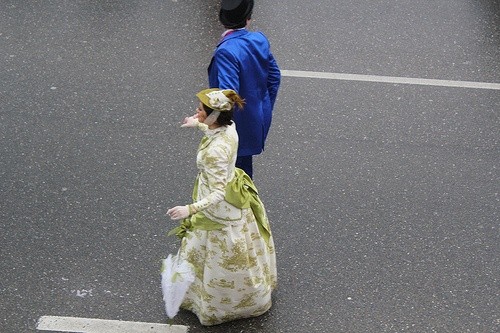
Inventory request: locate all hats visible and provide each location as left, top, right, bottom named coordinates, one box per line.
left=219, top=0, right=254, bottom=29
left=195, top=87, right=246, bottom=111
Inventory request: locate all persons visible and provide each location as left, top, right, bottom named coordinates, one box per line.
left=185, top=0, right=281, bottom=180
left=166, top=87, right=256, bottom=325
